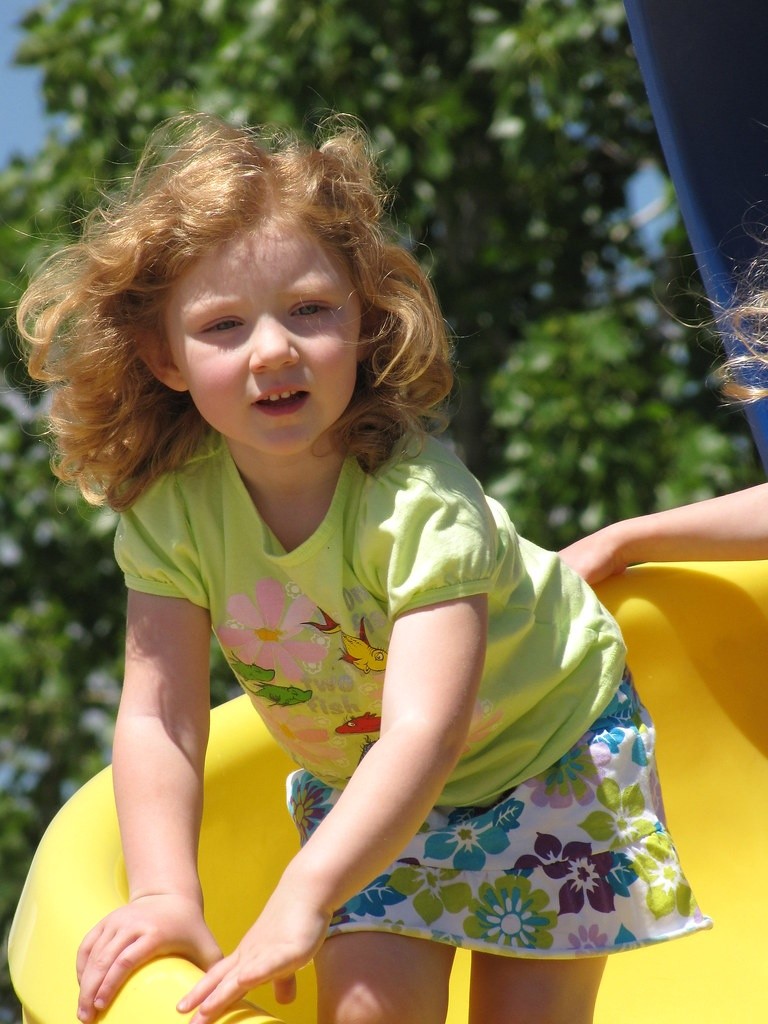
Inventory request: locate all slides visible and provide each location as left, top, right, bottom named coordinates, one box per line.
left=1, top=559, right=768, bottom=1024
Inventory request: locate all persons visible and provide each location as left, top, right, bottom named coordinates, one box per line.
left=13, top=111, right=712, bottom=1024
left=559, top=222, right=768, bottom=588
